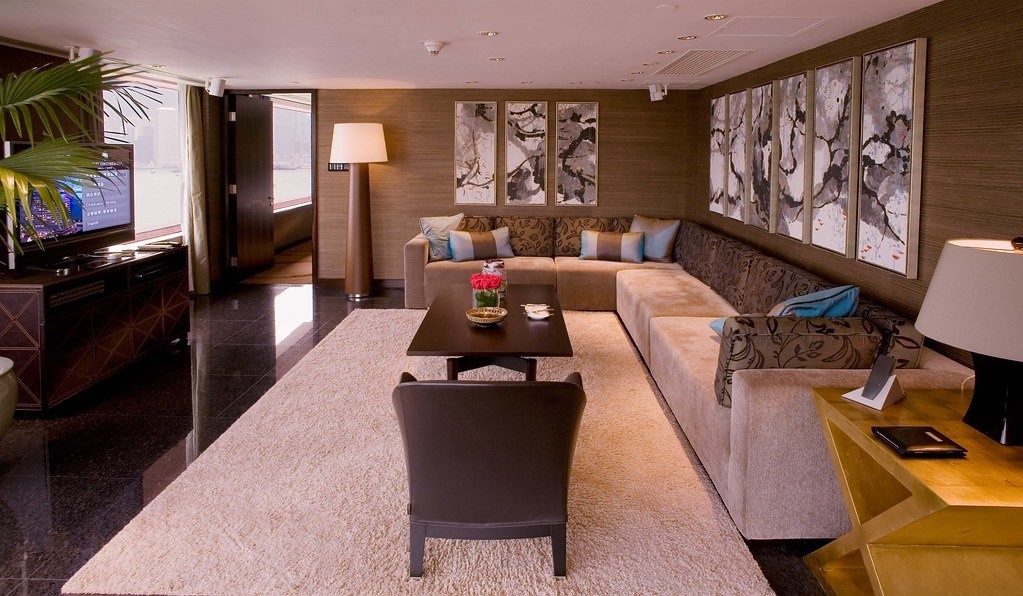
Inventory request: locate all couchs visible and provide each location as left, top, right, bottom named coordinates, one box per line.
left=403, top=215, right=976, bottom=541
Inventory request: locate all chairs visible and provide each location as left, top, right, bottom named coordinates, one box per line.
left=392, top=371, right=587, bottom=576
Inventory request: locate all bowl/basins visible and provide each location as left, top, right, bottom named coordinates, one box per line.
left=465, top=306, right=507, bottom=326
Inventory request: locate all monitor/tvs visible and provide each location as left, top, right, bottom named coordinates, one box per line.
left=3, top=141, right=135, bottom=272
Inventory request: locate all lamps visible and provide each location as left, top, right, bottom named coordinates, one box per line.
left=66, top=47, right=100, bottom=75
left=914, top=237, right=1023, bottom=447
left=329, top=122, right=390, bottom=303
left=204, top=77, right=226, bottom=98
left=649, top=83, right=667, bottom=102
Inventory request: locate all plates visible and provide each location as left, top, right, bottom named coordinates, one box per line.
left=525, top=303, right=550, bottom=319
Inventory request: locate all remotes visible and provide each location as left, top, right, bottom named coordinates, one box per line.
left=137, top=241, right=180, bottom=250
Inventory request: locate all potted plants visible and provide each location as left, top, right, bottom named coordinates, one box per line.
left=0, top=47, right=162, bottom=438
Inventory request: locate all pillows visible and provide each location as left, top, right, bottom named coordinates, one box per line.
left=629, top=214, right=681, bottom=263
left=449, top=226, right=515, bottom=261
left=765, top=283, right=860, bottom=318
left=577, top=229, right=644, bottom=263
left=708, top=313, right=759, bottom=337
left=418, top=212, right=466, bottom=262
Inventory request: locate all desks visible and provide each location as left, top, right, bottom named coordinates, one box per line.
left=803, top=383, right=1022, bottom=596
left=0, top=242, right=191, bottom=416
left=407, top=282, right=573, bottom=383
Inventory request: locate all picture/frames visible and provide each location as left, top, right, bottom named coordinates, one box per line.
left=855, top=37, right=926, bottom=281
left=774, top=70, right=809, bottom=246
left=706, top=80, right=780, bottom=233
left=809, top=58, right=861, bottom=258
left=504, top=101, right=548, bottom=206
left=454, top=100, right=497, bottom=207
left=555, top=101, right=599, bottom=207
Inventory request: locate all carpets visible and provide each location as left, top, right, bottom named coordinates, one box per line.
left=58, top=305, right=780, bottom=596
